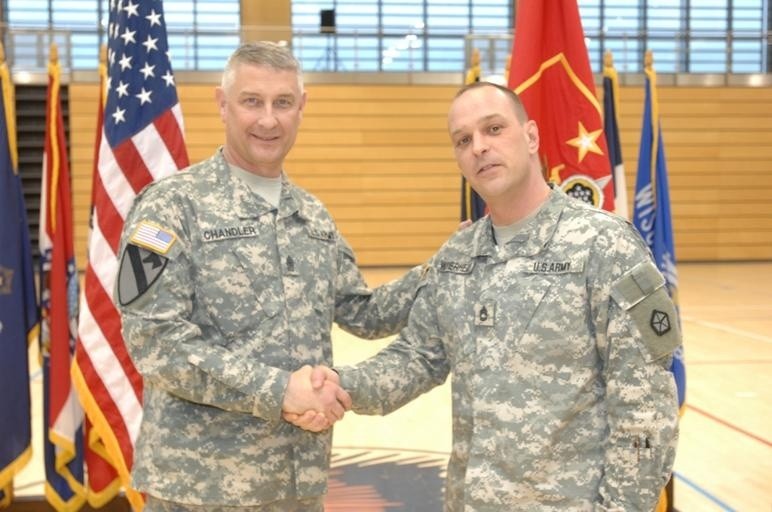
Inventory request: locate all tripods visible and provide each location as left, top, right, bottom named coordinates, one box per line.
left=313, top=34, right=347, bottom=71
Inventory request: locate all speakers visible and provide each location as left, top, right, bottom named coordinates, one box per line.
left=320, top=9, right=335, bottom=33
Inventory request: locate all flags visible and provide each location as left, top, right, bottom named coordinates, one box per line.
left=627, top=68, right=687, bottom=512
left=66, top=1, right=197, bottom=511
left=505, top=1, right=616, bottom=211
left=2, top=45, right=41, bottom=506
left=37, top=60, right=86, bottom=510
left=598, top=62, right=632, bottom=223
left=461, top=67, right=487, bottom=222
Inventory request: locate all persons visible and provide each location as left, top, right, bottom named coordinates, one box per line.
left=111, top=38, right=461, bottom=511
left=288, top=81, right=683, bottom=510
